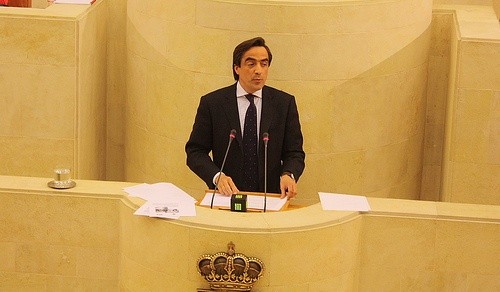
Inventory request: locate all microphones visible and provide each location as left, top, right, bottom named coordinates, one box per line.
left=211, top=129, right=236, bottom=209
left=262, top=133, right=269, bottom=212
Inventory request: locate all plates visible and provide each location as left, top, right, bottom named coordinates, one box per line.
left=48, top=180, right=75, bottom=188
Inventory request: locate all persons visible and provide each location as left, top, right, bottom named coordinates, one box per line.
left=184, top=37, right=305, bottom=200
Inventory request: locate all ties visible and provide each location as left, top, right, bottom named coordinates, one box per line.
left=242, top=93, right=260, bottom=190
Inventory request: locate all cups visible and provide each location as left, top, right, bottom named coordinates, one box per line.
left=54, top=167, right=71, bottom=186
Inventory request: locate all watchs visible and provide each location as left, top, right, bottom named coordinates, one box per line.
left=281, top=172, right=294, bottom=179
